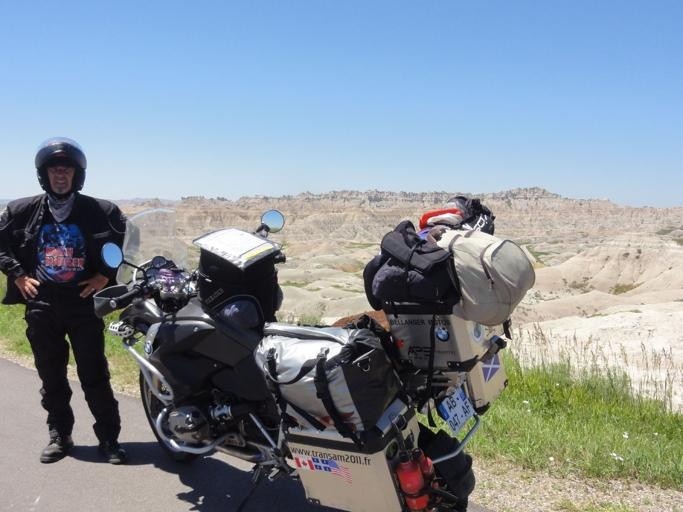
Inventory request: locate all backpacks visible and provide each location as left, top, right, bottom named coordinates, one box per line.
left=426, top=221, right=538, bottom=328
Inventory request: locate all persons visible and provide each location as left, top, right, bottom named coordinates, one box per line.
left=0, top=137, right=139, bottom=464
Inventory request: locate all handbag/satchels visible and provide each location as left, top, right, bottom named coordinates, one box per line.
left=362, top=218, right=463, bottom=318
left=442, top=195, right=497, bottom=236
left=252, top=312, right=421, bottom=444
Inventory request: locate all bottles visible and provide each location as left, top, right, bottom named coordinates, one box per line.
left=396, top=452, right=428, bottom=510
left=413, top=449, right=440, bottom=491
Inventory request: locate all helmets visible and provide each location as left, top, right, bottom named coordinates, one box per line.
left=34, top=136, right=88, bottom=195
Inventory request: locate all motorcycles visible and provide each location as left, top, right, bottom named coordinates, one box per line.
left=90, top=208, right=511, bottom=512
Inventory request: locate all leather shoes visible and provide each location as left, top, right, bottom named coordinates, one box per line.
left=38, top=431, right=76, bottom=464
left=99, top=437, right=130, bottom=466
